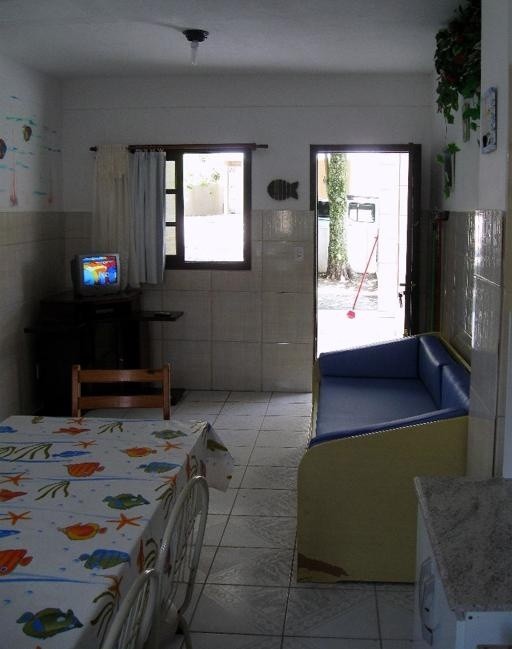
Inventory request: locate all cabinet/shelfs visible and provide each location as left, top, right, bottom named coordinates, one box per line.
left=40, top=288, right=185, bottom=407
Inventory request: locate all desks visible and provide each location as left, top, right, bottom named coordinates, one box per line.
left=2, top=410, right=211, bottom=649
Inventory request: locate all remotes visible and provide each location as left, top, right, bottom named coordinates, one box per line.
left=154, top=311, right=170, bottom=316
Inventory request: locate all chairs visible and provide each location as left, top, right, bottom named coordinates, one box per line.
left=101, top=569, right=161, bottom=647
left=348, top=195, right=378, bottom=222
left=155, top=471, right=210, bottom=649
left=69, top=363, right=173, bottom=421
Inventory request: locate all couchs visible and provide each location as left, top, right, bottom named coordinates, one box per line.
left=295, top=333, right=474, bottom=586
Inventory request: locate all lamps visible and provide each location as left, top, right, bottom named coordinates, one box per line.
left=183, top=29, right=210, bottom=67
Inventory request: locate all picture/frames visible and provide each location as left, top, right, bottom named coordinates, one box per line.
left=479, top=87, right=497, bottom=154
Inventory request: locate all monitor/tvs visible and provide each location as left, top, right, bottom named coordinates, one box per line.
left=73, top=253, right=120, bottom=300
